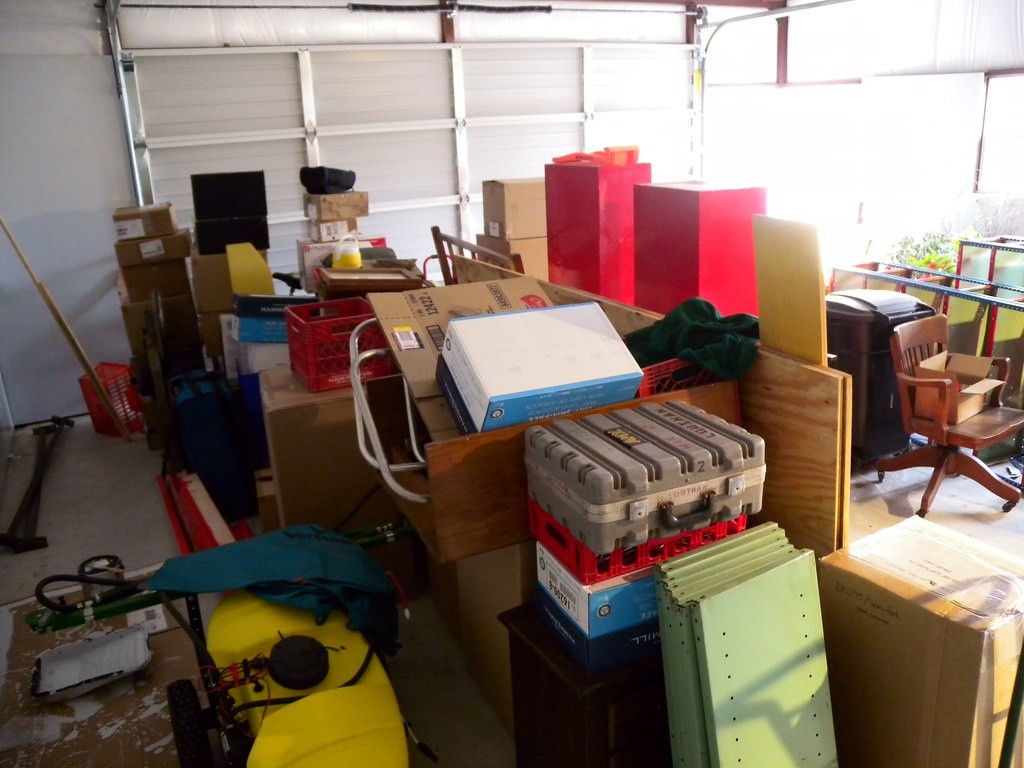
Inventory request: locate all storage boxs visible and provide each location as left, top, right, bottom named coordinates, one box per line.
left=77, top=147, right=1024, bottom=768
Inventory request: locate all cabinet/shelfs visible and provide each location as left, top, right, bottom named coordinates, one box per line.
left=497, top=601, right=674, bottom=768
left=830, top=259, right=1024, bottom=411
left=955, top=235, right=1024, bottom=291
left=359, top=371, right=742, bottom=563
left=634, top=179, right=768, bottom=316
left=545, top=161, right=652, bottom=305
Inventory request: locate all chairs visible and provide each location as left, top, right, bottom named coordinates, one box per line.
left=876, top=314, right=1024, bottom=520
left=430, top=226, right=517, bottom=286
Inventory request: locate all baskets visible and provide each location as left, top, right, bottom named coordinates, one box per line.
left=284, top=296, right=401, bottom=392
left=79, top=362, right=146, bottom=436
